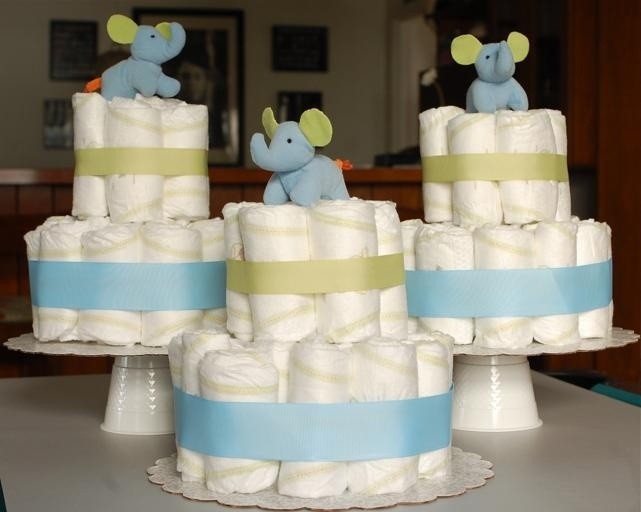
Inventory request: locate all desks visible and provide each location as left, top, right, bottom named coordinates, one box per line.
left=1, top=366, right=641, bottom=512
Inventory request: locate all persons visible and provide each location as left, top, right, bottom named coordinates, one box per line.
left=170, top=46, right=225, bottom=147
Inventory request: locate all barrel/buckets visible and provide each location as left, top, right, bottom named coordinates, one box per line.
left=100, top=355, right=175, bottom=437
left=453, top=356, right=542, bottom=434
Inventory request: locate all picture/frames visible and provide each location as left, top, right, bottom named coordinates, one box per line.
left=50, top=7, right=246, bottom=169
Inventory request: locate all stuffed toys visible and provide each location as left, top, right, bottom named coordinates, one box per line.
left=81, top=11, right=190, bottom=104
left=247, top=106, right=356, bottom=205
left=449, top=30, right=534, bottom=112
